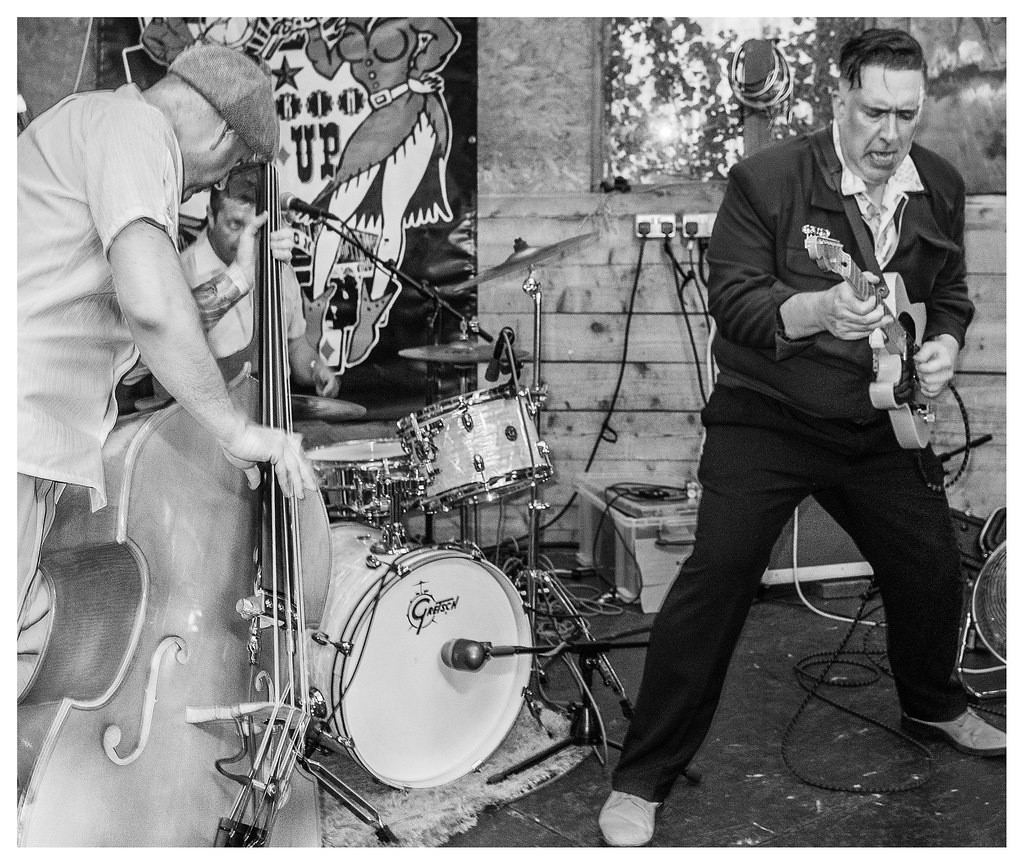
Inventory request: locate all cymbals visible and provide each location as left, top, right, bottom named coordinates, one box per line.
left=281, top=393, right=369, bottom=424
left=453, top=231, right=603, bottom=293
left=398, top=342, right=531, bottom=365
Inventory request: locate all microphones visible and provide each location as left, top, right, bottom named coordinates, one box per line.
left=441, top=639, right=487, bottom=673
left=279, top=192, right=341, bottom=222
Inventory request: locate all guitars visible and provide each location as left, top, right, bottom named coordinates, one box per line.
left=802, top=230, right=942, bottom=450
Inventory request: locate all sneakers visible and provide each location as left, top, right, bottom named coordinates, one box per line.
left=599, top=789, right=662, bottom=848
left=901, top=707, right=1006, bottom=758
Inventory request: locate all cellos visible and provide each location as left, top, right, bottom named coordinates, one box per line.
left=17, top=156, right=331, bottom=848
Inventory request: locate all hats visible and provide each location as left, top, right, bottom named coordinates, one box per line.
left=168, top=45, right=278, bottom=161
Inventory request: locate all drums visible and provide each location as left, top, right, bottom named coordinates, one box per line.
left=303, top=522, right=535, bottom=788
left=302, top=437, right=427, bottom=524
left=392, top=384, right=555, bottom=519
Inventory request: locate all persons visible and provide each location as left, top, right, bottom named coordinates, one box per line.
left=177, top=160, right=341, bottom=411
left=596, top=26, right=1007, bottom=846
left=17, top=46, right=324, bottom=706
